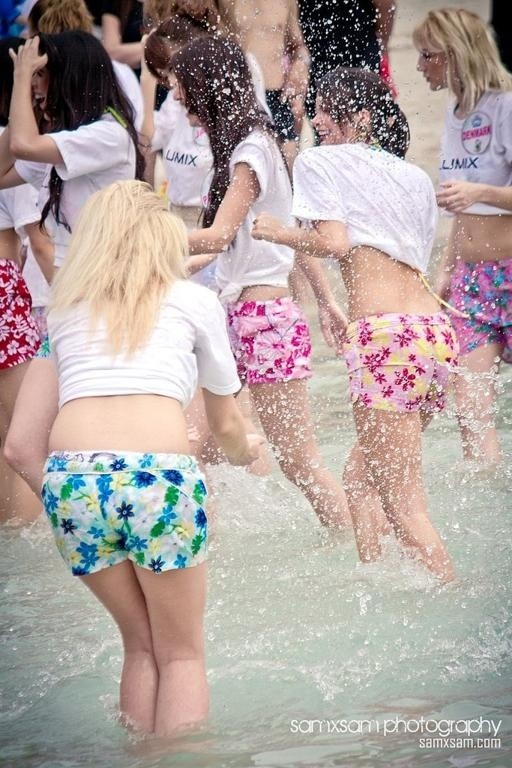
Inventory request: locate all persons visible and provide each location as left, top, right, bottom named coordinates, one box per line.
left=250, top=66, right=465, bottom=589
left=0, top=176, right=264, bottom=749
left=410, top=5, right=511, bottom=478
left=1, top=0, right=414, bottom=534
